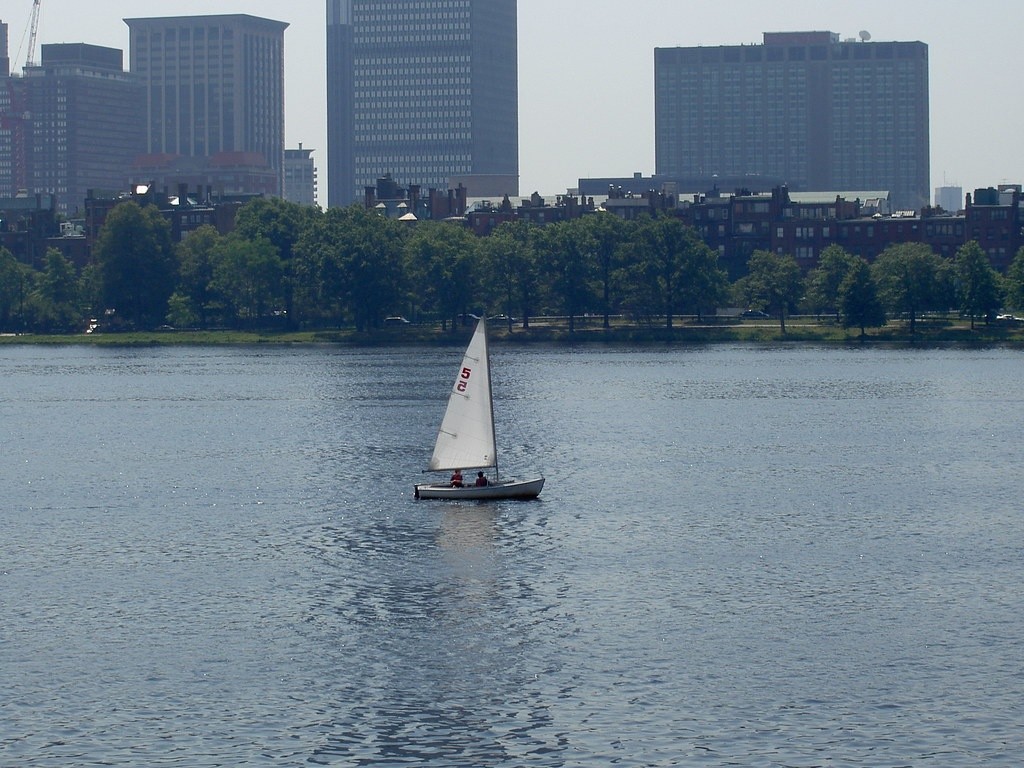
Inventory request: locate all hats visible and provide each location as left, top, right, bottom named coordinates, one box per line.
left=478, top=471, right=484, bottom=474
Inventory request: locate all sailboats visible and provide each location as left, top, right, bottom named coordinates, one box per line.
left=414, top=312, right=546, bottom=501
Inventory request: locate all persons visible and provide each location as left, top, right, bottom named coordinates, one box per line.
left=476, top=471, right=488, bottom=487
left=450, top=470, right=464, bottom=489
